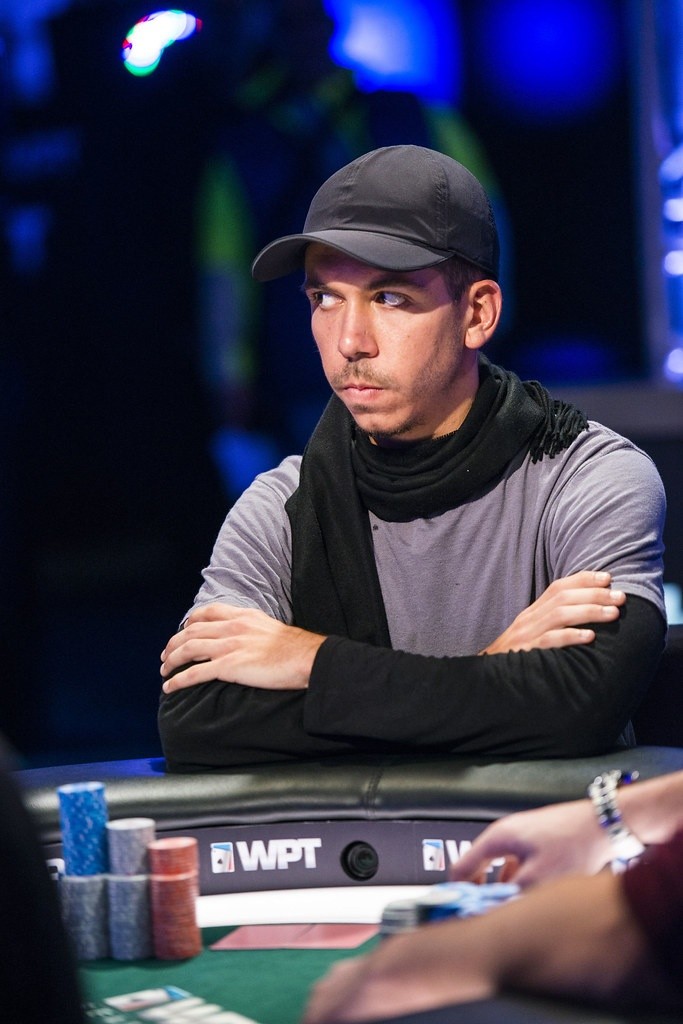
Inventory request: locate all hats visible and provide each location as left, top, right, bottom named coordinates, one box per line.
left=250, top=143, right=500, bottom=284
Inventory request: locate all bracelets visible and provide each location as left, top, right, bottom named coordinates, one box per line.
left=586, top=769, right=647, bottom=860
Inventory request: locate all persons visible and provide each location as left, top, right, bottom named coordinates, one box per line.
left=195, top=1, right=513, bottom=481
left=156, top=142, right=667, bottom=766
left=305, top=766, right=682, bottom=1024
